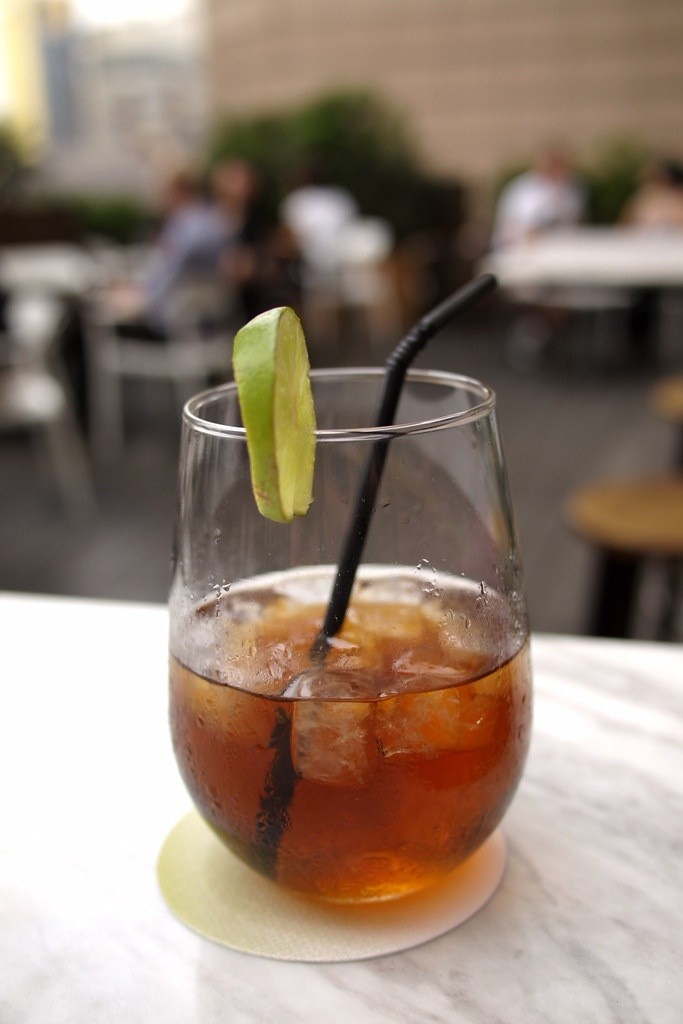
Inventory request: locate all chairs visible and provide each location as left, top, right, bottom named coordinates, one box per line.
left=559, top=380, right=683, bottom=644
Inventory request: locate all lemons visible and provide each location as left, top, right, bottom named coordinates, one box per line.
left=232, top=305, right=317, bottom=524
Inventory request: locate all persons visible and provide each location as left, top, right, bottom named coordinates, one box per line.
left=490, top=138, right=682, bottom=374
left=114, top=138, right=420, bottom=385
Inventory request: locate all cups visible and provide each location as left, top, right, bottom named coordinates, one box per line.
left=164, top=368, right=532, bottom=913
